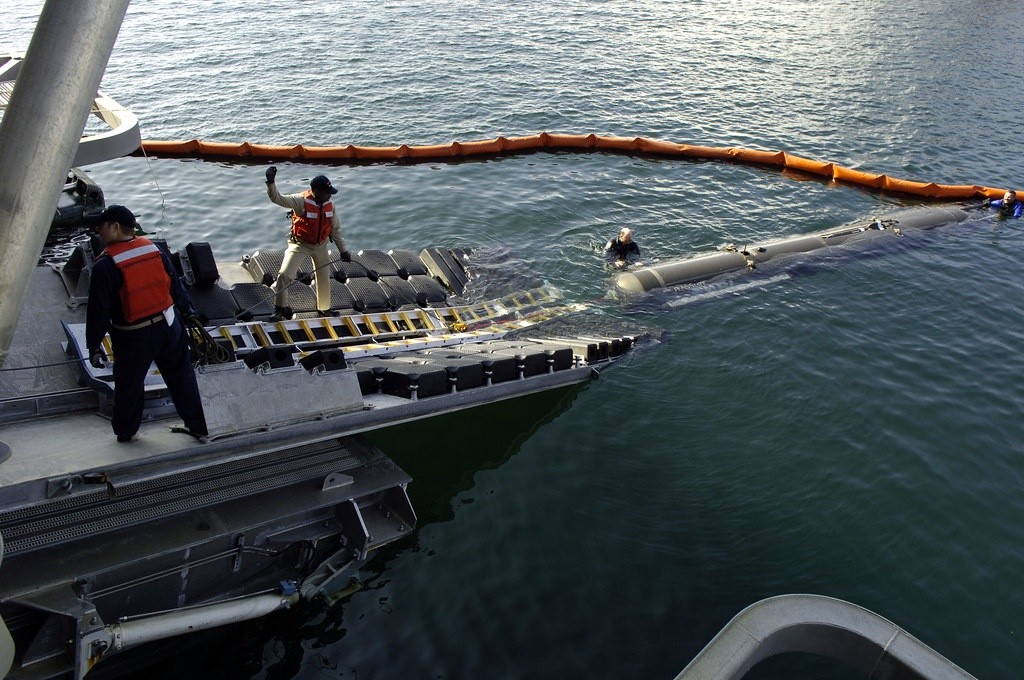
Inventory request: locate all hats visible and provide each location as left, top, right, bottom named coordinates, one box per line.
left=88, top=205, right=136, bottom=227
left=310, top=175, right=338, bottom=194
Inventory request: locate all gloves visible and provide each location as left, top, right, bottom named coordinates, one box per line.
left=341, top=251, right=351, bottom=263
left=179, top=304, right=197, bottom=327
left=89, top=346, right=108, bottom=369
left=266, top=166, right=277, bottom=183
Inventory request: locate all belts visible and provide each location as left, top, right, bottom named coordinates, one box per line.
left=110, top=314, right=165, bottom=330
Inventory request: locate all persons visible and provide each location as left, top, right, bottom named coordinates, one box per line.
left=86, top=205, right=208, bottom=443
left=991, top=190, right=1021, bottom=217
left=265, top=166, right=351, bottom=322
left=605, top=228, right=641, bottom=265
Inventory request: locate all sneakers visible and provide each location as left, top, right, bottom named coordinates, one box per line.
left=269, top=305, right=287, bottom=322
left=318, top=309, right=340, bottom=317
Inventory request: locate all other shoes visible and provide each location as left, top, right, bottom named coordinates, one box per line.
left=118, top=432, right=132, bottom=442
